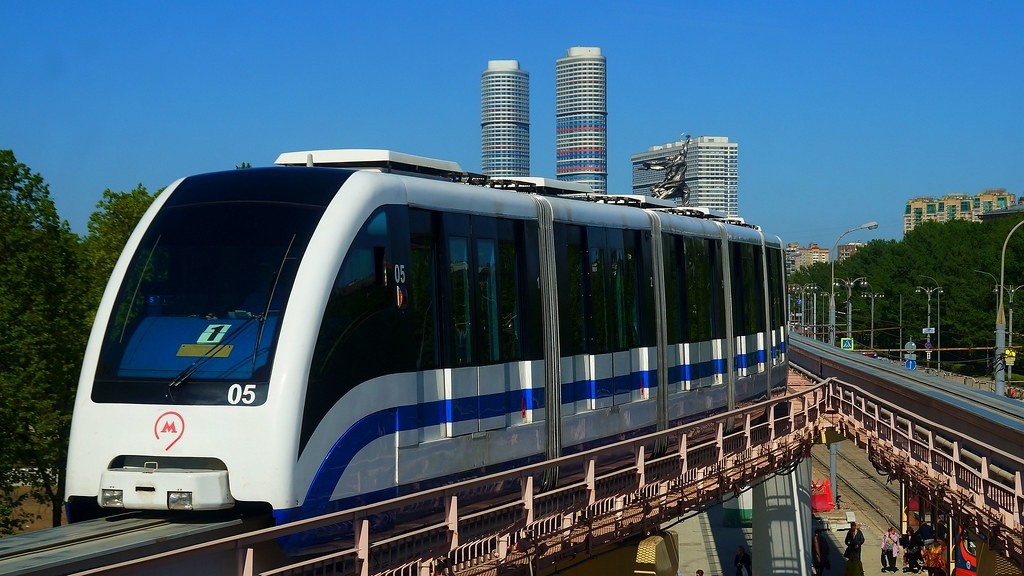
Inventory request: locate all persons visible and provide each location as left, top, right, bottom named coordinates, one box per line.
left=845, top=522, right=922, bottom=574
left=696, top=544, right=752, bottom=576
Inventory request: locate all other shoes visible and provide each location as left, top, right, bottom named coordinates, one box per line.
left=889, top=567, right=894, bottom=570
left=918, top=567, right=922, bottom=573
left=904, top=568, right=912, bottom=571
left=882, top=567, right=887, bottom=570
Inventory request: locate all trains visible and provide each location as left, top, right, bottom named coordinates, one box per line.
left=61, top=150, right=793, bottom=522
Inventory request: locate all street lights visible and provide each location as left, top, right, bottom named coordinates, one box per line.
left=787, top=282, right=839, bottom=343
left=991, top=283, right=1024, bottom=396
left=831, top=276, right=868, bottom=345
left=860, top=290, right=885, bottom=350
left=920, top=274, right=941, bottom=372
left=974, top=269, right=1006, bottom=397
left=915, top=285, right=943, bottom=369
left=826, top=222, right=880, bottom=347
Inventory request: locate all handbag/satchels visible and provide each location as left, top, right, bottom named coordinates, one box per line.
left=843, top=544, right=857, bottom=558
